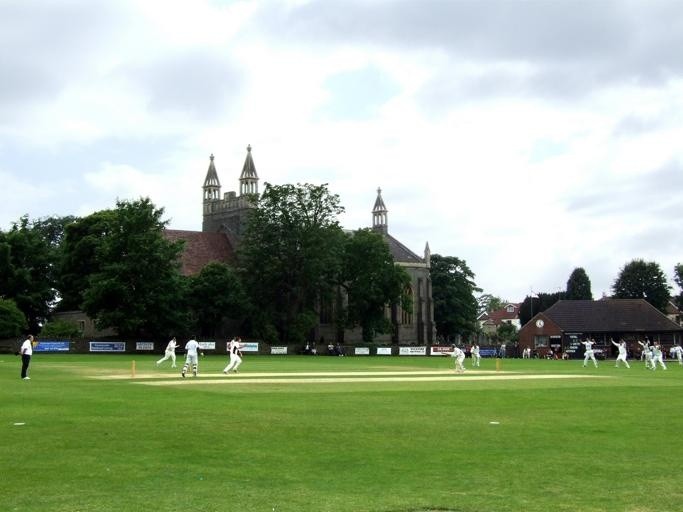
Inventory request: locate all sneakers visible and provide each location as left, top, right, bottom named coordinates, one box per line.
left=182, top=371, right=197, bottom=378
left=23, top=377, right=31, bottom=379
left=223, top=369, right=238, bottom=374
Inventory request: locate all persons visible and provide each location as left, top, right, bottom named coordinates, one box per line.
left=181, top=334, right=201, bottom=378
left=546, top=347, right=570, bottom=360
left=450, top=343, right=466, bottom=372
left=156, top=336, right=181, bottom=368
left=670, top=343, right=683, bottom=364
left=522, top=347, right=531, bottom=359
left=581, top=338, right=599, bottom=368
left=611, top=337, right=629, bottom=369
left=635, top=339, right=668, bottom=371
left=328, top=341, right=344, bottom=356
left=303, top=341, right=318, bottom=355
left=223, top=336, right=242, bottom=374
left=19, top=335, right=34, bottom=380
left=470, top=342, right=480, bottom=367
left=500, top=341, right=507, bottom=358
